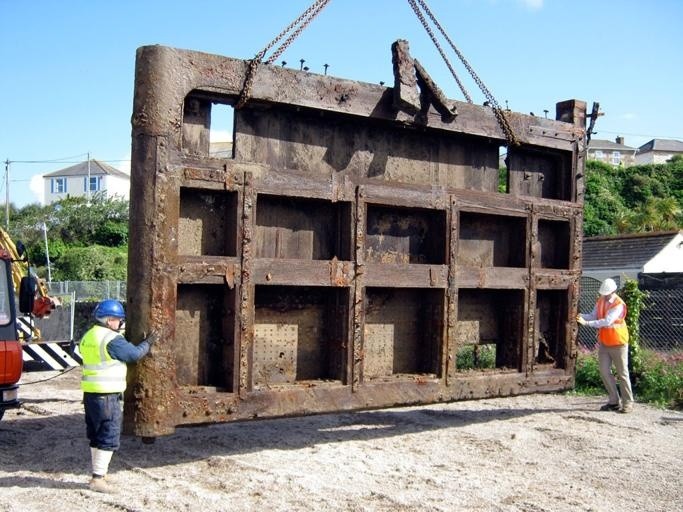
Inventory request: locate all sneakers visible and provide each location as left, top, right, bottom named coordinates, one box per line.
left=88, top=476, right=114, bottom=494
left=601, top=403, right=621, bottom=410
left=622, top=402, right=633, bottom=412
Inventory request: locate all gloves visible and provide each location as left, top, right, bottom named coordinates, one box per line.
left=576, top=315, right=588, bottom=326
left=141, top=331, right=158, bottom=346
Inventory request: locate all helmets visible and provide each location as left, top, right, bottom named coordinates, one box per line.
left=599, top=279, right=617, bottom=295
left=93, top=300, right=124, bottom=318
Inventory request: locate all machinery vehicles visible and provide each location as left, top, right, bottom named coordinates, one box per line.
left=1, top=228, right=56, bottom=421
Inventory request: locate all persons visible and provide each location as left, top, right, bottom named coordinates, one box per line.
left=574, top=278, right=634, bottom=413
left=79, top=300, right=160, bottom=495
